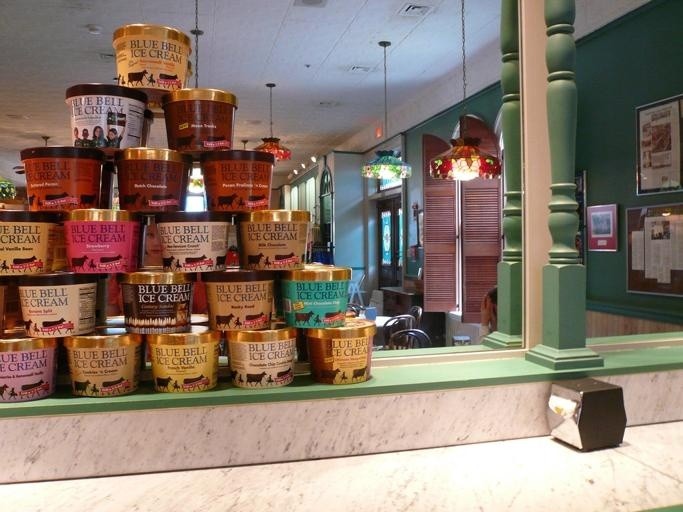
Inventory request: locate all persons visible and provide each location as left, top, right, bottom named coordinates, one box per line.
left=72, top=127, right=82, bottom=147
left=80, top=128, right=95, bottom=147
left=105, top=120, right=127, bottom=148
left=478, top=286, right=497, bottom=341
left=91, top=126, right=108, bottom=148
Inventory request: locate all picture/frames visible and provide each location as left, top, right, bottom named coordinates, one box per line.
left=587, top=204, right=618, bottom=252
left=625, top=202, right=683, bottom=298
left=635, top=93, right=683, bottom=197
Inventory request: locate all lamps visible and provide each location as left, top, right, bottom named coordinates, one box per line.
left=360, top=40, right=412, bottom=180
left=430, top=0, right=502, bottom=179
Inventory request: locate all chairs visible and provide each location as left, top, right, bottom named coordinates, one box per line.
left=381, top=306, right=433, bottom=349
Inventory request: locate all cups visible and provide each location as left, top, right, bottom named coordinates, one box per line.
left=363, top=306, right=379, bottom=323
